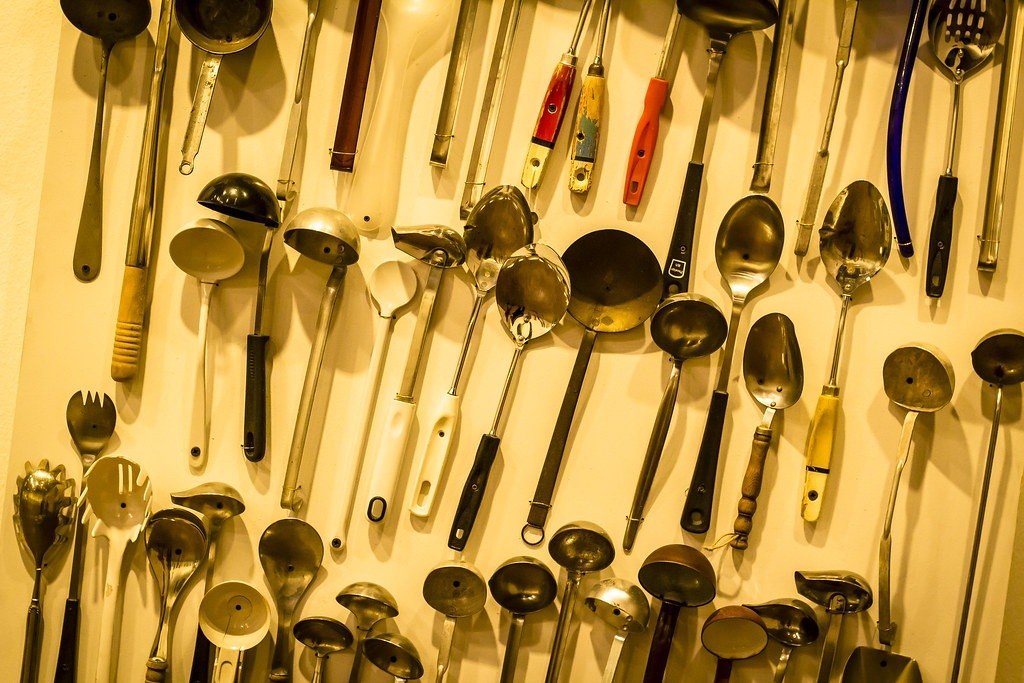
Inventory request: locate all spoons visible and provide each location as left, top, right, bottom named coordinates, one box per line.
left=726, top=312, right=804, bottom=550
left=802, top=180, right=894, bottom=524
left=407, top=185, right=535, bottom=521
left=257, top=518, right=324, bottom=683
left=448, top=241, right=570, bottom=550
left=328, top=259, right=418, bottom=550
left=678, top=194, right=784, bottom=536
left=145, top=508, right=209, bottom=683
